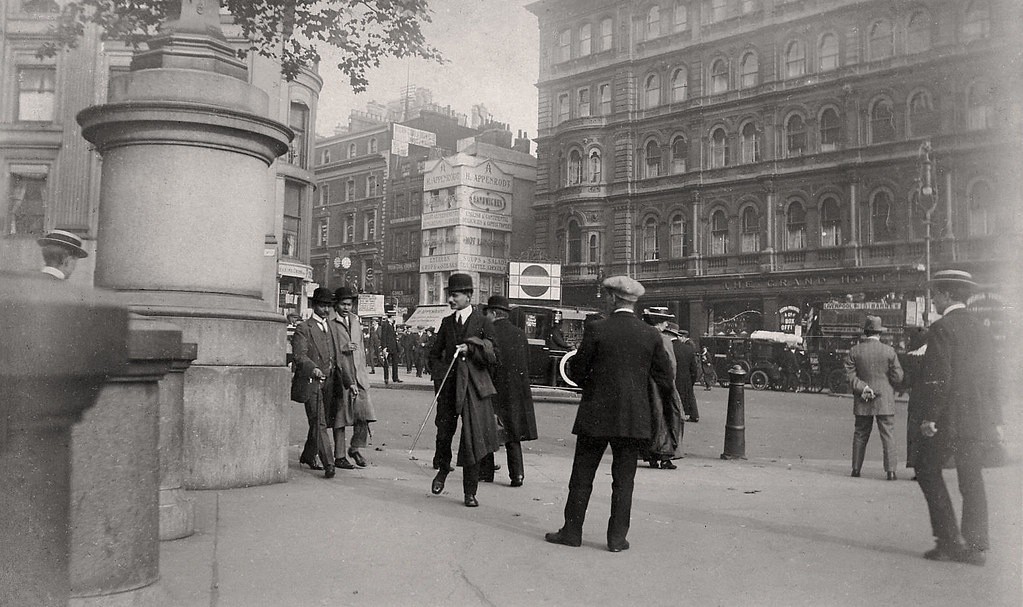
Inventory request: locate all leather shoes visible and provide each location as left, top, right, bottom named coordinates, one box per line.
left=431, top=470, right=447, bottom=493
left=300, top=452, right=368, bottom=477
left=465, top=494, right=478, bottom=506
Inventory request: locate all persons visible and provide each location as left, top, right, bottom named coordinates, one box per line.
left=286, top=311, right=435, bottom=386
left=842, top=314, right=905, bottom=480
left=478, top=294, right=538, bottom=486
left=328, top=289, right=377, bottom=469
left=906, top=268, right=1002, bottom=569
left=290, top=286, right=343, bottom=478
left=430, top=275, right=501, bottom=508
left=536, top=275, right=710, bottom=553
left=782, top=344, right=828, bottom=393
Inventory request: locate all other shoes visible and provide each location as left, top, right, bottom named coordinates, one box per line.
left=370, top=367, right=434, bottom=386
left=544, top=532, right=580, bottom=547
left=888, top=472, right=897, bottom=480
left=511, top=476, right=523, bottom=486
left=955, top=545, right=986, bottom=565
left=495, top=465, right=500, bottom=470
left=610, top=540, right=629, bottom=553
left=851, top=470, right=860, bottom=477
left=924, top=535, right=967, bottom=559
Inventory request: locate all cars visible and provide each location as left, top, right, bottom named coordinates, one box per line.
left=696, top=331, right=924, bottom=395
left=512, top=304, right=601, bottom=383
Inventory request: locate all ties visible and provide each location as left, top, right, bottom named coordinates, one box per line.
left=343, top=317, right=349, bottom=326
left=318, top=321, right=326, bottom=333
left=456, top=314, right=462, bottom=335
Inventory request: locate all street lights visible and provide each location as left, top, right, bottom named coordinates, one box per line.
left=913, top=142, right=935, bottom=336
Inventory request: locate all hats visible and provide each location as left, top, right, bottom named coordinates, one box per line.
left=374, top=312, right=434, bottom=332
left=860, top=316, right=888, bottom=331
left=308, top=287, right=337, bottom=303
left=603, top=276, right=646, bottom=303
left=642, top=306, right=675, bottom=317
left=925, top=269, right=978, bottom=288
left=665, top=321, right=682, bottom=335
left=332, top=288, right=358, bottom=298
left=444, top=273, right=477, bottom=291
left=483, top=296, right=512, bottom=312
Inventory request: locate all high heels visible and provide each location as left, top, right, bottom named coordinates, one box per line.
left=661, top=460, right=676, bottom=469
left=649, top=460, right=658, bottom=467
